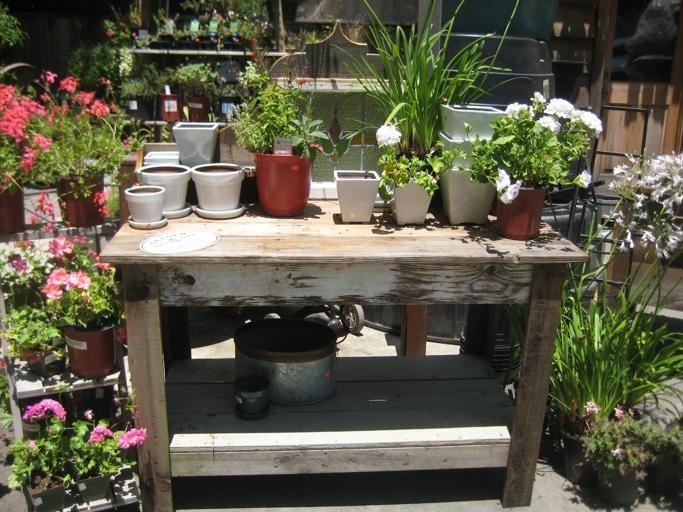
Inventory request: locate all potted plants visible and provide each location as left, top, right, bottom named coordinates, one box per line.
left=500, top=264, right=682, bottom=483
left=129, top=62, right=247, bottom=122
left=230, top=62, right=334, bottom=217
left=1, top=306, right=64, bottom=376
left=134, top=10, right=264, bottom=52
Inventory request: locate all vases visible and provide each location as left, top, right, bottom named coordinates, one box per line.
left=191, top=163, right=245, bottom=219
left=383, top=180, right=437, bottom=226
left=124, top=186, right=167, bottom=230
left=596, top=467, right=642, bottom=507
left=27, top=461, right=67, bottom=499
left=0, top=167, right=105, bottom=236
left=134, top=165, right=192, bottom=218
left=496, top=185, right=549, bottom=240
left=437, top=103, right=505, bottom=225
left=334, top=170, right=380, bottom=224
left=64, top=322, right=115, bottom=379
left=172, top=122, right=218, bottom=167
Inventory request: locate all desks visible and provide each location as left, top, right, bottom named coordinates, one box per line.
left=99, top=201, right=590, bottom=511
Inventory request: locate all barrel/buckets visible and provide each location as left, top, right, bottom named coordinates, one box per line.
left=236, top=320, right=336, bottom=404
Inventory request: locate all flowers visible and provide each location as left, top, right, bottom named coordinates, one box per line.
left=464, top=92, right=603, bottom=205
left=375, top=125, right=467, bottom=202
left=41, top=256, right=123, bottom=329
left=0, top=71, right=140, bottom=182
left=6, top=398, right=75, bottom=488
left=576, top=399, right=657, bottom=487
left=1, top=236, right=96, bottom=284
left=562, top=148, right=683, bottom=318
left=70, top=410, right=148, bottom=477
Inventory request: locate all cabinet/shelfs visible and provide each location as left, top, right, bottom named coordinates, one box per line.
left=110, top=0, right=306, bottom=142
left=5, top=342, right=146, bottom=511
